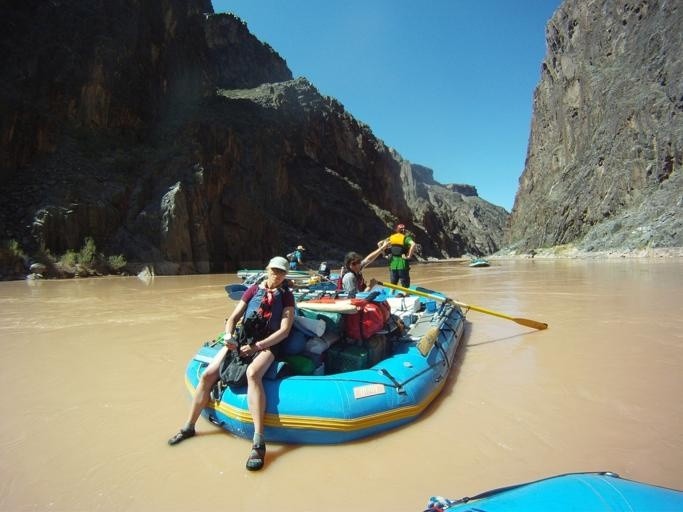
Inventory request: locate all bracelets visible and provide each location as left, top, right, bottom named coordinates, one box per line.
left=256, top=342, right=262, bottom=351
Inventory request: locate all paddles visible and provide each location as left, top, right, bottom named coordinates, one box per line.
left=416, top=305, right=457, bottom=356
left=224, top=286, right=354, bottom=300
left=376, top=281, right=548, bottom=331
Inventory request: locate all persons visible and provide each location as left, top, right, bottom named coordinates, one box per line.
left=168, top=256, right=295, bottom=470
left=286, top=245, right=306, bottom=270
left=342, top=241, right=393, bottom=295
left=377, top=224, right=416, bottom=296
left=318, top=261, right=330, bottom=282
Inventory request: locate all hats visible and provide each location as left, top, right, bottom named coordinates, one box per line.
left=296, top=246, right=306, bottom=251
left=397, top=224, right=406, bottom=233
left=265, top=256, right=289, bottom=274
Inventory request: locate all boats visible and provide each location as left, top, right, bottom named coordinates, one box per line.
left=235, top=267, right=348, bottom=302
left=184, top=269, right=465, bottom=445
left=468, top=258, right=489, bottom=267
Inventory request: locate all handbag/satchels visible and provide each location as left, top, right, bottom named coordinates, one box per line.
left=284, top=295, right=421, bottom=376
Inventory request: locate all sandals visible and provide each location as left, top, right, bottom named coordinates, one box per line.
left=168, top=428, right=195, bottom=445
left=246, top=440, right=266, bottom=471
